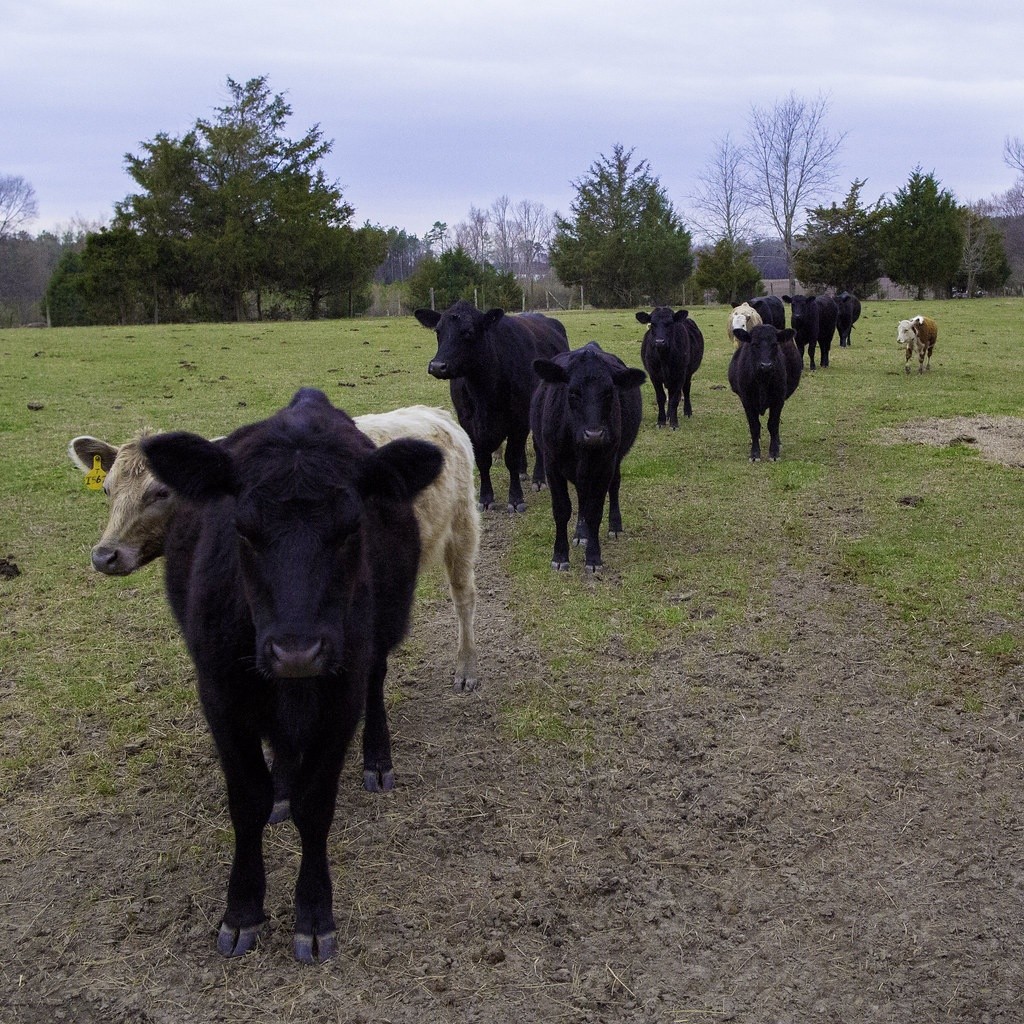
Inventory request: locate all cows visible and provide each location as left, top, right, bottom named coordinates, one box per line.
left=897, top=315, right=938, bottom=375
left=726, top=291, right=861, bottom=369
left=529, top=341, right=647, bottom=573
left=636, top=308, right=704, bottom=431
left=414, top=299, right=572, bottom=512
left=727, top=324, right=804, bottom=462
left=68, top=388, right=482, bottom=963
